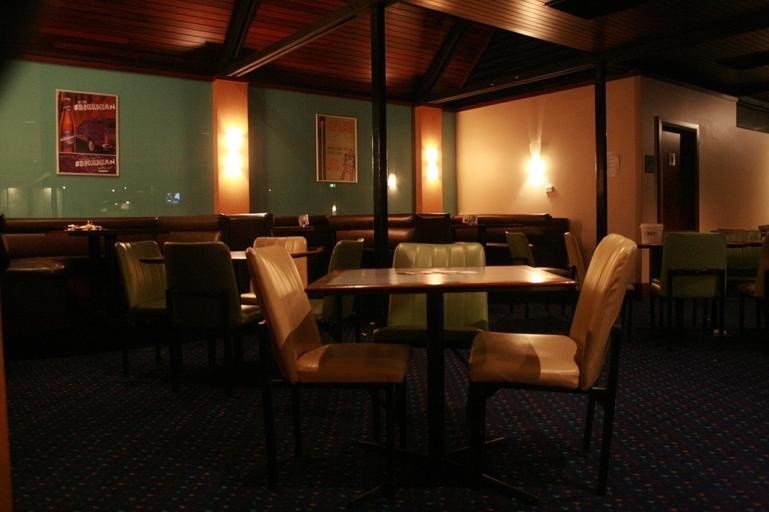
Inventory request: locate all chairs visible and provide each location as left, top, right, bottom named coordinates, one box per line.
left=240, top=233, right=306, bottom=304
left=309, top=235, right=366, bottom=345
left=560, top=231, right=635, bottom=344
left=737, top=235, right=767, bottom=339
left=244, top=245, right=416, bottom=465
left=650, top=230, right=725, bottom=335
left=162, top=241, right=264, bottom=401
left=112, top=240, right=169, bottom=377
left=462, top=232, right=641, bottom=493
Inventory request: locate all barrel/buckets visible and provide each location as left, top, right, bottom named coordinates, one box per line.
left=639, top=223, right=664, bottom=245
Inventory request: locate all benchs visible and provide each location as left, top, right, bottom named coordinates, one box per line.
left=1, top=209, right=572, bottom=346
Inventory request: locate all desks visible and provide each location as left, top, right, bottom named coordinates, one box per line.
left=637, top=240, right=764, bottom=335
left=139, top=251, right=324, bottom=389
left=304, top=265, right=577, bottom=491
